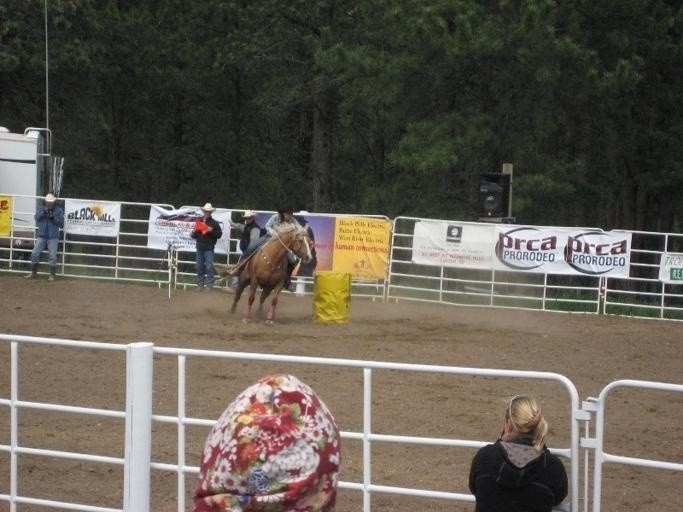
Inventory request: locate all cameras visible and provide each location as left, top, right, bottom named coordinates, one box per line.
left=44, top=208, right=54, bottom=219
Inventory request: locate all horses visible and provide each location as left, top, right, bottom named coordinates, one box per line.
left=227, top=221, right=313, bottom=326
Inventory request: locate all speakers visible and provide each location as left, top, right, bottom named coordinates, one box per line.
left=477, top=172, right=510, bottom=217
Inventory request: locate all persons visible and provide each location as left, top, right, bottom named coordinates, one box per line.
left=226, top=201, right=301, bottom=292
left=226, top=210, right=261, bottom=292
left=188, top=201, right=221, bottom=293
left=22, top=194, right=64, bottom=281
left=193, top=374, right=342, bottom=511
left=295, top=215, right=317, bottom=276
left=468, top=392, right=568, bottom=512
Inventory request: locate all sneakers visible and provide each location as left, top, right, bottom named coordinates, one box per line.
left=23, top=274, right=39, bottom=280
left=49, top=275, right=57, bottom=281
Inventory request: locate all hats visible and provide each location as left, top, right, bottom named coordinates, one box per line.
left=201, top=203, right=215, bottom=211
left=276, top=202, right=293, bottom=213
left=45, top=194, right=56, bottom=203
left=243, top=210, right=255, bottom=217
